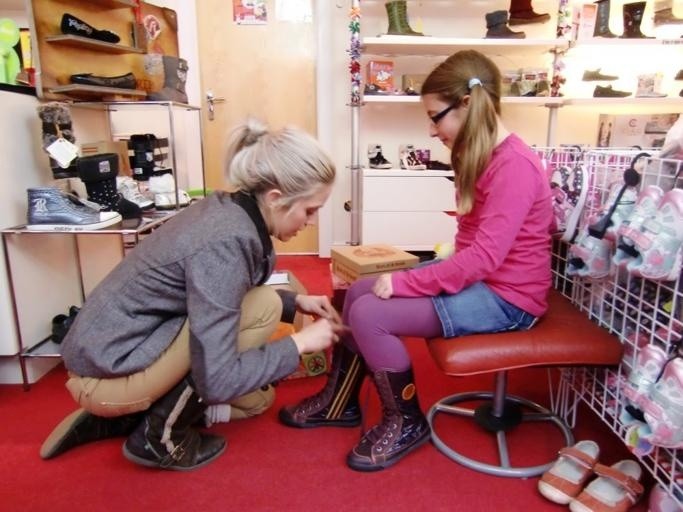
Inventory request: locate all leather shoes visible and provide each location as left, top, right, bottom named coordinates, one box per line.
left=61, top=13, right=120, bottom=42
left=363, top=84, right=389, bottom=94
left=594, top=85, right=630, bottom=97
left=583, top=70, right=617, bottom=81
left=72, top=73, right=136, bottom=89
left=49, top=305, right=80, bottom=343
left=515, top=77, right=551, bottom=96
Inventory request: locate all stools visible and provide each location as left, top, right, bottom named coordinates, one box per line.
left=426, top=288, right=626, bottom=479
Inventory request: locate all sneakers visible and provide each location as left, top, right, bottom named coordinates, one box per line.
left=538, top=440, right=646, bottom=512
left=547, top=164, right=683, bottom=281
left=622, top=344, right=683, bottom=448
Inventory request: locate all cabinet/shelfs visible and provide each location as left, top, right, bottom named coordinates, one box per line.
left=564, top=36, right=683, bottom=106
left=343, top=0, right=570, bottom=252
left=0, top=101, right=206, bottom=392
left=26, top=0, right=148, bottom=101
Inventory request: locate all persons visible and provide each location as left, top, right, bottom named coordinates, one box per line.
left=278, top=49, right=553, bottom=471
left=39, top=118, right=336, bottom=469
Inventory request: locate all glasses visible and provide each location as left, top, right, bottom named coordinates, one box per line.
left=429, top=104, right=458, bottom=127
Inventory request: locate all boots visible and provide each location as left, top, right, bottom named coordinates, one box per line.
left=278, top=343, right=366, bottom=428
left=399, top=145, right=426, bottom=169
left=486, top=0, right=549, bottom=38
left=368, top=145, right=392, bottom=169
left=594, top=1, right=681, bottom=38
left=26, top=102, right=192, bottom=231
left=123, top=377, right=228, bottom=470
left=147, top=55, right=189, bottom=104
left=347, top=365, right=431, bottom=473
left=386, top=1, right=420, bottom=34
left=636, top=74, right=668, bottom=99
left=40, top=408, right=147, bottom=460
